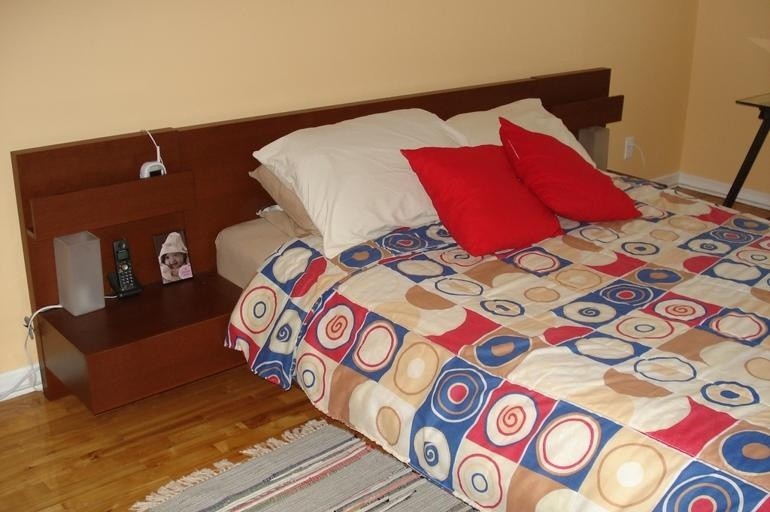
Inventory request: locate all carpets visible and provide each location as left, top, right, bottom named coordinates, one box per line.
left=128, top=416, right=476, bottom=512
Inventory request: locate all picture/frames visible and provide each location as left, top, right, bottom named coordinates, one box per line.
left=150, top=229, right=193, bottom=284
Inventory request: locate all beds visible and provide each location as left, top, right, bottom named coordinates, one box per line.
left=215, top=171, right=770, bottom=512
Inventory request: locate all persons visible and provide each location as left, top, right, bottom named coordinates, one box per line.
left=158, top=233, right=189, bottom=285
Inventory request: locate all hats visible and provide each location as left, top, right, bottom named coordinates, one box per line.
left=158, top=232, right=188, bottom=264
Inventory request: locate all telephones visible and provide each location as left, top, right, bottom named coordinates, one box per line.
left=108, top=239, right=144, bottom=297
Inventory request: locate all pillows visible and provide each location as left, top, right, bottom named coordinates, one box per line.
left=400, top=141, right=565, bottom=259
left=445, top=94, right=600, bottom=172
left=497, top=117, right=642, bottom=239
left=250, top=105, right=470, bottom=259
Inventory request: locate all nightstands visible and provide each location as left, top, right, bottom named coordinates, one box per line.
left=39, top=274, right=246, bottom=415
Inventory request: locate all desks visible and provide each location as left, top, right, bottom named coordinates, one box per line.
left=722, top=92, right=770, bottom=208
